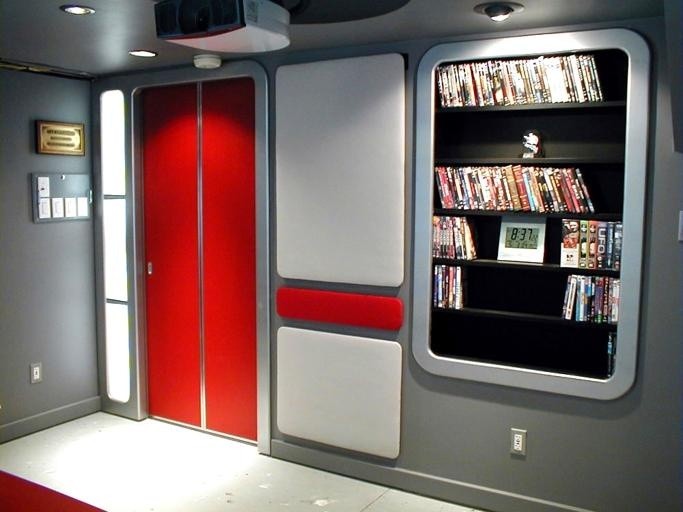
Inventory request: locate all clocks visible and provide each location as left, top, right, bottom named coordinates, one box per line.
left=494, top=211, right=548, bottom=263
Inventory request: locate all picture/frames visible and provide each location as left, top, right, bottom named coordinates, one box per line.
left=33, top=119, right=85, bottom=157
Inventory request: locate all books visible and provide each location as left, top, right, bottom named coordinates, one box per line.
left=560, top=220, right=622, bottom=270
left=433, top=266, right=470, bottom=310
left=563, top=275, right=621, bottom=324
left=435, top=166, right=599, bottom=212
left=433, top=216, right=479, bottom=260
left=437, top=55, right=607, bottom=107
left=605, top=334, right=617, bottom=377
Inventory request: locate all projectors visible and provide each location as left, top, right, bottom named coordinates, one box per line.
left=154, top=0, right=291, bottom=53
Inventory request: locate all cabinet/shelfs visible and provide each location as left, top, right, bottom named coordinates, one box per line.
left=431, top=101, right=626, bottom=330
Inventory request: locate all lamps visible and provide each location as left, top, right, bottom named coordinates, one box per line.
left=472, top=2, right=525, bottom=22
